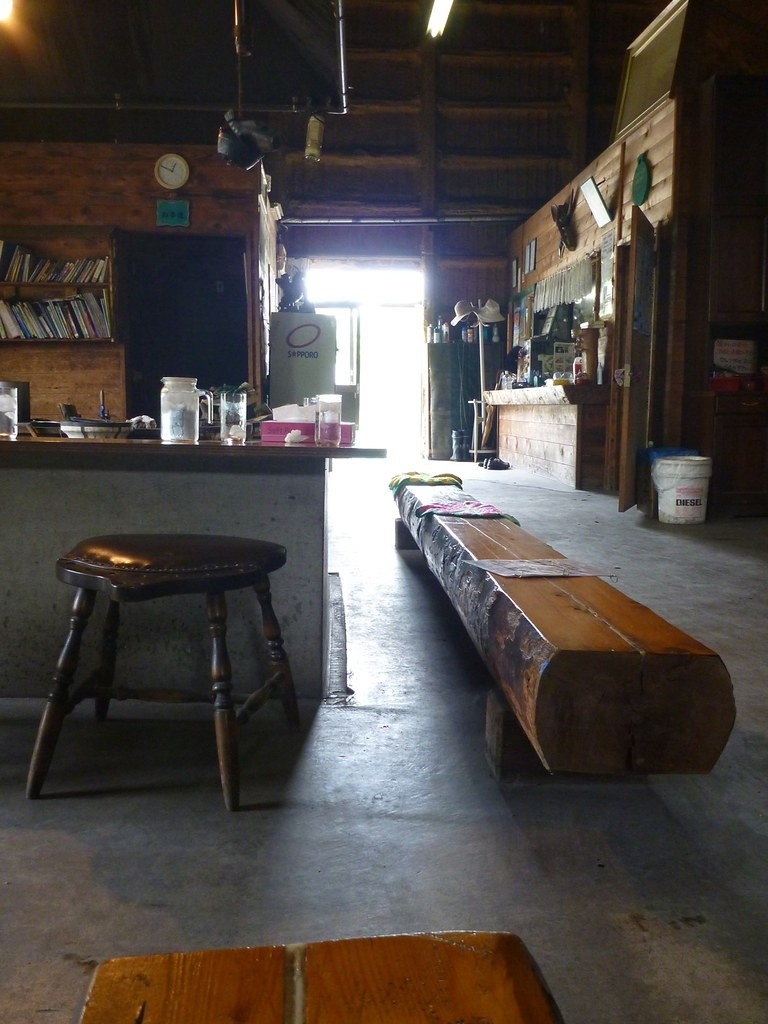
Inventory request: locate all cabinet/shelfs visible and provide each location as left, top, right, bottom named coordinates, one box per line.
left=0, top=223, right=124, bottom=343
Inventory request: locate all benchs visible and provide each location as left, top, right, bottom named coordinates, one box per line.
left=395, top=474, right=736, bottom=790
left=82, top=928, right=566, bottom=1024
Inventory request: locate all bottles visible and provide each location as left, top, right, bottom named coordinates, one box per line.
left=437, top=314, right=443, bottom=330
left=433, top=327, right=441, bottom=344
left=596, top=362, right=603, bottom=384
left=502, top=371, right=513, bottom=390
left=427, top=324, right=432, bottom=344
left=442, top=322, right=450, bottom=344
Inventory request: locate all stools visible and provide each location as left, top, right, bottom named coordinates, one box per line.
left=22, top=534, right=297, bottom=812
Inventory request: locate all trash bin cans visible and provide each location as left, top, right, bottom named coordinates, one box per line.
left=451, top=429, right=470, bottom=462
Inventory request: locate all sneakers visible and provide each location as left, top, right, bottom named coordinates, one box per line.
left=484, top=457, right=510, bottom=470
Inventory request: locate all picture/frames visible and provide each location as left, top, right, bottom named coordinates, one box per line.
left=579, top=176, right=613, bottom=229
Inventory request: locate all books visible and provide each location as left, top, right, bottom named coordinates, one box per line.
left=0, top=240, right=111, bottom=340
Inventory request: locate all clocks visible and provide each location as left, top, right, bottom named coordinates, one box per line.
left=154, top=154, right=189, bottom=190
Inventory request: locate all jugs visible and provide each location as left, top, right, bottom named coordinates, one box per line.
left=159, top=376, right=213, bottom=445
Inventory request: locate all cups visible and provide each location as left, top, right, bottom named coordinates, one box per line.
left=304, top=398, right=317, bottom=408
left=220, top=392, right=247, bottom=445
left=315, top=394, right=342, bottom=447
left=0, top=386, right=18, bottom=441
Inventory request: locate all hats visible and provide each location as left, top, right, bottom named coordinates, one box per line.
left=451, top=300, right=476, bottom=326
left=478, top=299, right=505, bottom=322
